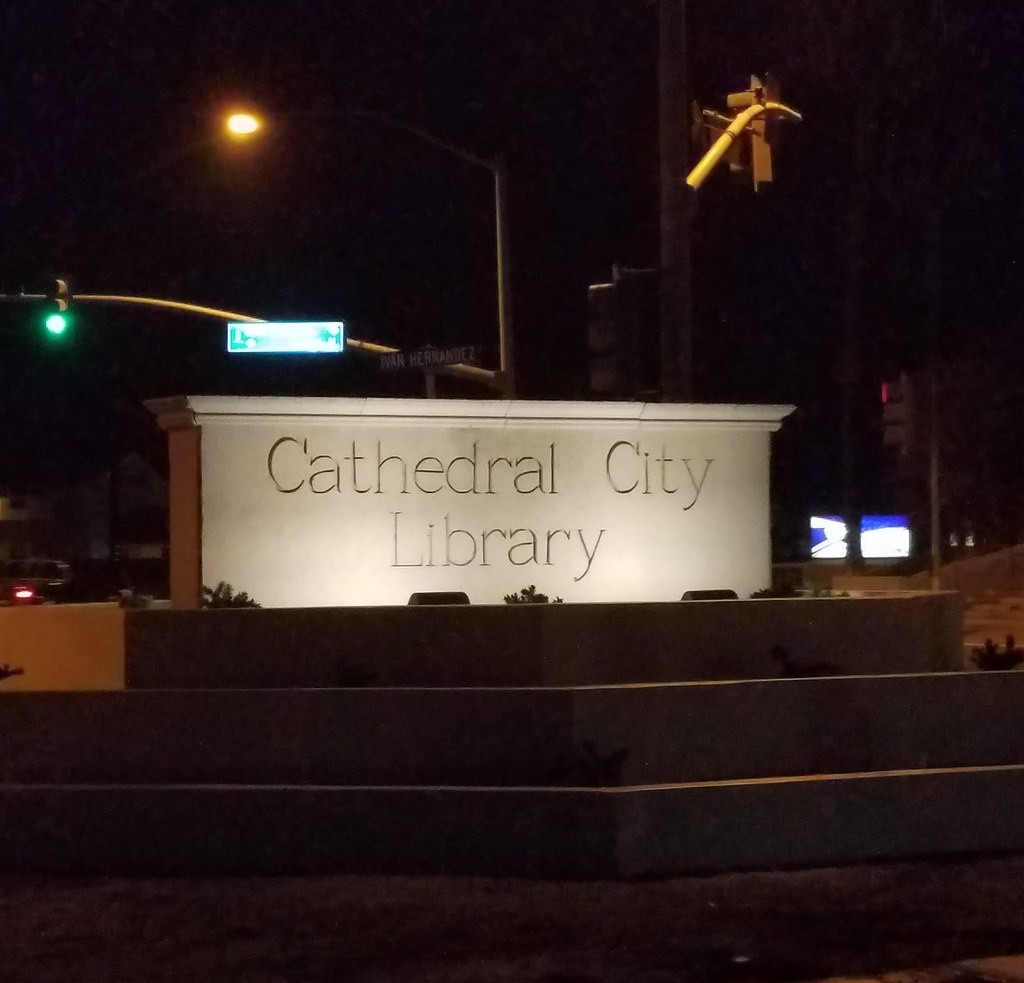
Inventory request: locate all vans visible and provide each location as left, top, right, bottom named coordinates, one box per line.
left=0, top=559, right=73, bottom=606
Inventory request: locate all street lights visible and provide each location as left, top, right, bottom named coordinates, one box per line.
left=206, top=97, right=516, bottom=397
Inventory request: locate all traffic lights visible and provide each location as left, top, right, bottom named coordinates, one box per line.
left=41, top=279, right=72, bottom=342
left=881, top=371, right=909, bottom=456
